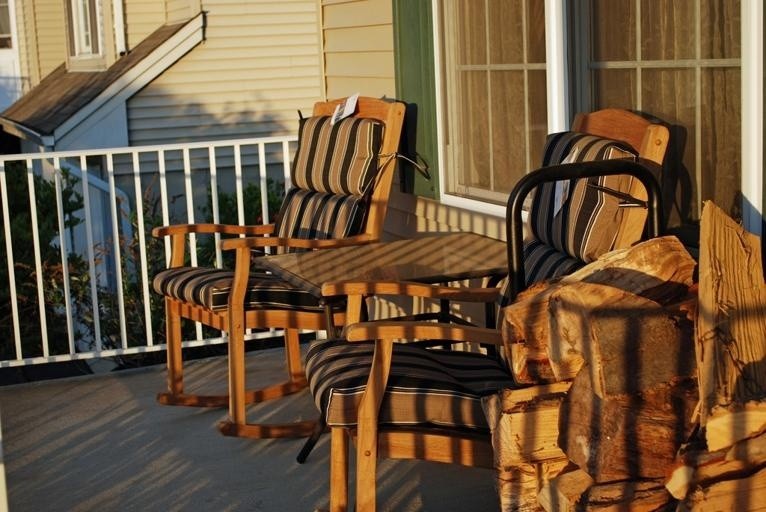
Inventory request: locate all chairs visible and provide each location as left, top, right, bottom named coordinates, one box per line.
left=149, top=92, right=411, bottom=442
left=300, top=106, right=688, bottom=512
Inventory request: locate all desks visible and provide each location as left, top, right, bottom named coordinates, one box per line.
left=249, top=226, right=525, bottom=463
